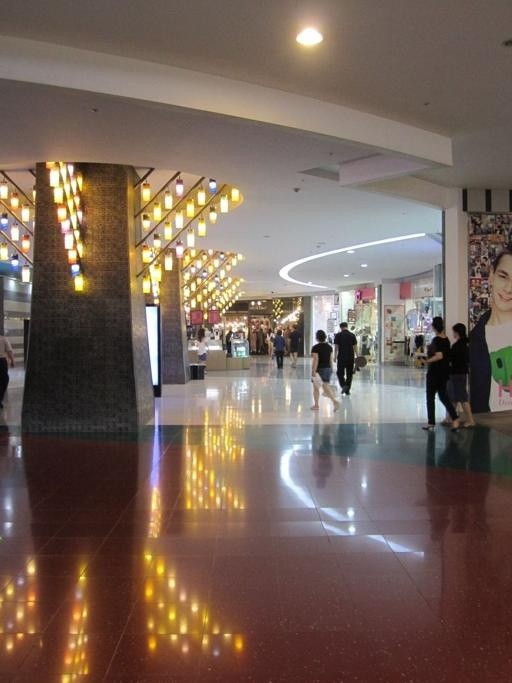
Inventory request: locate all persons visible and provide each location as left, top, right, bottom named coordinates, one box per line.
left=468, top=211, right=512, bottom=414
left=310, top=329, right=339, bottom=411
left=311, top=407, right=337, bottom=488
left=1, top=406, right=17, bottom=476
left=221, top=323, right=301, bottom=369
left=334, top=320, right=359, bottom=394
left=360, top=327, right=372, bottom=362
left=0, top=335, right=17, bottom=407
left=439, top=322, right=477, bottom=429
left=421, top=315, right=463, bottom=433
left=335, top=394, right=358, bottom=467
left=196, top=328, right=210, bottom=374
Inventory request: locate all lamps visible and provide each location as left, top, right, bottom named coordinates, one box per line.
left=46, top=160, right=84, bottom=297
left=137, top=167, right=247, bottom=318
left=1, top=172, right=34, bottom=282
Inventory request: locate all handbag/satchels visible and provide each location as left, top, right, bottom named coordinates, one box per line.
left=312, top=372, right=323, bottom=389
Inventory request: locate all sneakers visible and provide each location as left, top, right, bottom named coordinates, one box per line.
left=341, top=385, right=350, bottom=395
left=422, top=419, right=476, bottom=430
left=312, top=406, right=319, bottom=410
